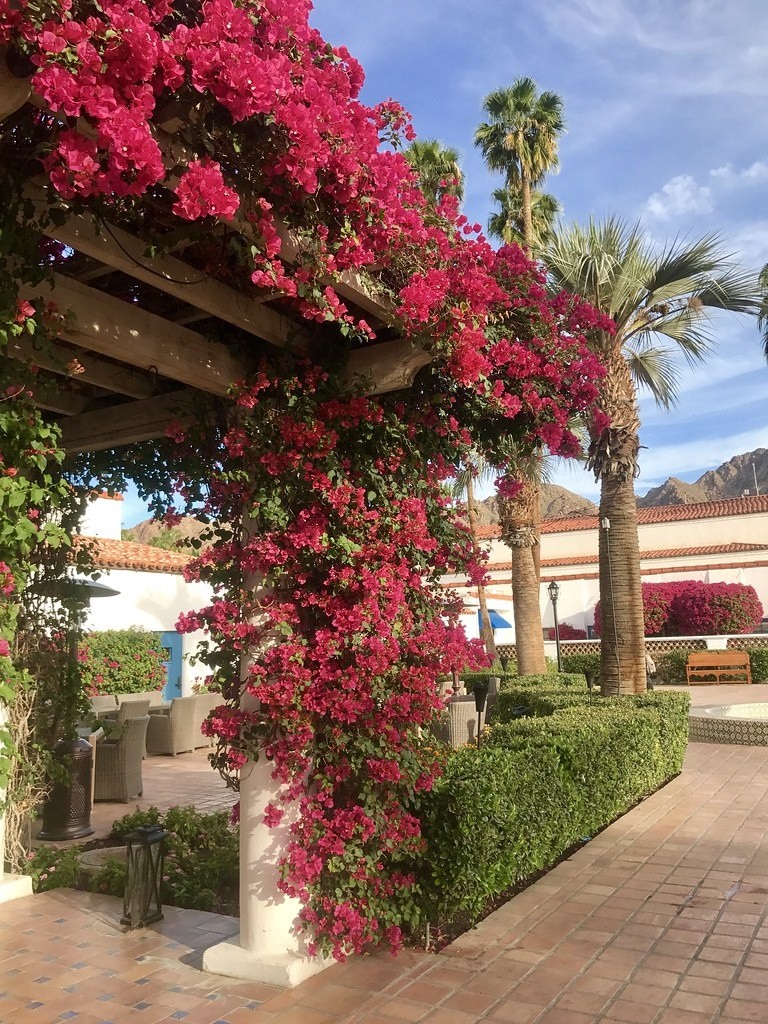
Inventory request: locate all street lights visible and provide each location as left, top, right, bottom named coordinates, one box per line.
left=547, top=579, right=564, bottom=673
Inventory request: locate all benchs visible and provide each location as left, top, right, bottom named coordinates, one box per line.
left=685, top=651, right=753, bottom=687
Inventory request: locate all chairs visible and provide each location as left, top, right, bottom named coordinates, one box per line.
left=91, top=692, right=230, bottom=804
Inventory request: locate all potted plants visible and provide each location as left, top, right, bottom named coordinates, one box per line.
left=15, top=494, right=96, bottom=842
